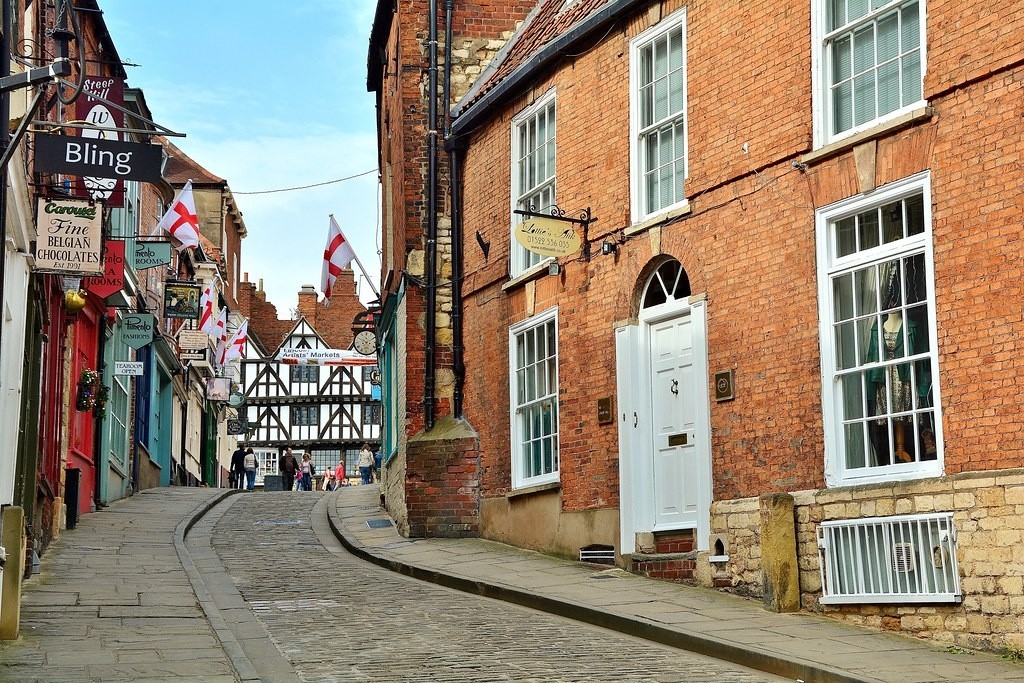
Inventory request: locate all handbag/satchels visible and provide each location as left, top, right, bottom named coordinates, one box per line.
left=227, top=470, right=237, bottom=483
left=321, top=476, right=325, bottom=482
left=371, top=461, right=375, bottom=468
left=310, top=464, right=315, bottom=475
left=324, top=472, right=332, bottom=479
left=254, top=454, right=259, bottom=468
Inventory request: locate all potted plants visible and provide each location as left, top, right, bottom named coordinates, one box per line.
left=264, top=474, right=283, bottom=491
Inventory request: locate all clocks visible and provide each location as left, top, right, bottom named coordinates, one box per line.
left=351, top=327, right=377, bottom=355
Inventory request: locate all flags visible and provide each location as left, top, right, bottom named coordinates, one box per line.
left=321, top=219, right=354, bottom=301
left=199, top=286, right=213, bottom=335
left=160, top=184, right=199, bottom=248
left=213, top=311, right=227, bottom=366
left=228, top=320, right=247, bottom=360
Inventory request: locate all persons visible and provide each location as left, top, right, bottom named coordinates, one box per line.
left=865, top=306, right=928, bottom=426
left=244, top=447, right=259, bottom=490
left=279, top=447, right=301, bottom=491
left=294, top=443, right=376, bottom=491
left=230, top=443, right=248, bottom=489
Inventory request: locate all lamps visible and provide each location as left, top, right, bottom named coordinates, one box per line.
left=601, top=241, right=620, bottom=257
left=548, top=257, right=562, bottom=277
left=31, top=269, right=91, bottom=311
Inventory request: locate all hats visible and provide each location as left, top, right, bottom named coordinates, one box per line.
left=240, top=443, right=245, bottom=449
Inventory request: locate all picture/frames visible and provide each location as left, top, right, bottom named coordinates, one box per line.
left=164, top=284, right=201, bottom=320
left=206, top=377, right=232, bottom=401
